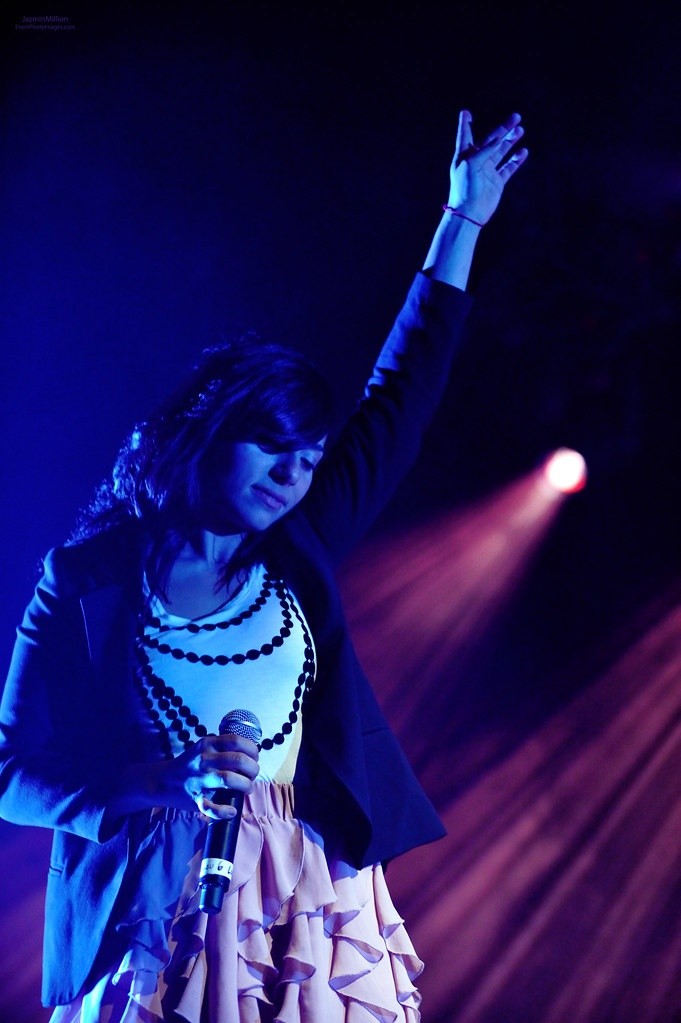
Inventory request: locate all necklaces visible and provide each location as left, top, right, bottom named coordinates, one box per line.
left=131, top=564, right=316, bottom=756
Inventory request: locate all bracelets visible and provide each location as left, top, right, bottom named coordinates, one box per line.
left=443, top=203, right=485, bottom=228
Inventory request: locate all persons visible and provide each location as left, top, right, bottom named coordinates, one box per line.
left=1, top=110, right=528, bottom=1023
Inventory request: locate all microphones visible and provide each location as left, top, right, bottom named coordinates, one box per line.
left=196, top=709, right=264, bottom=914
